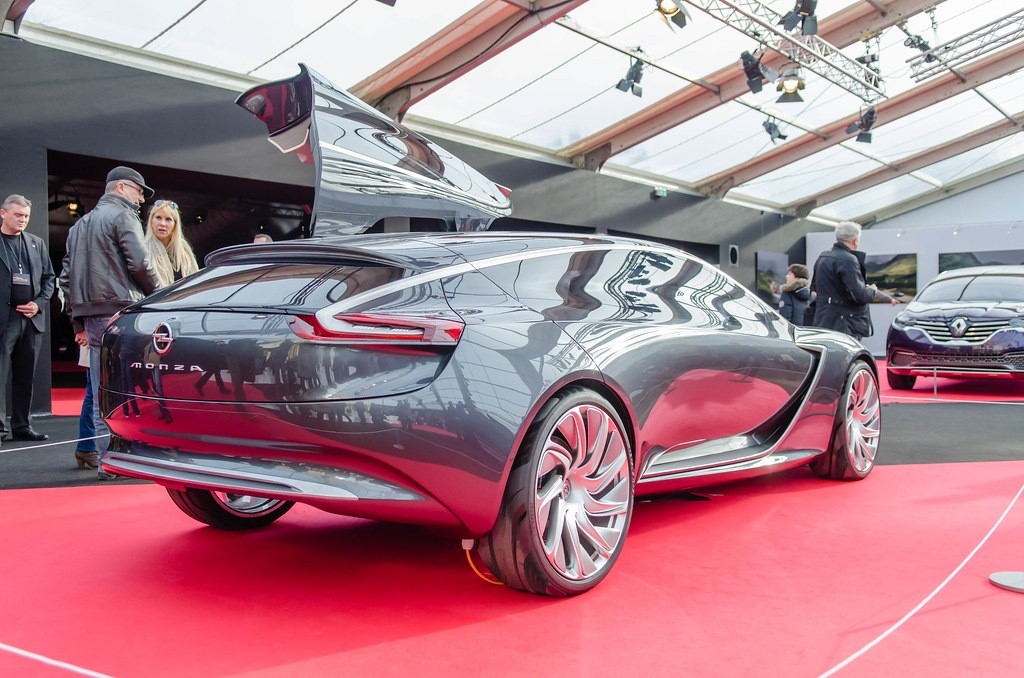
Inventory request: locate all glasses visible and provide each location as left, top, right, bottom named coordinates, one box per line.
left=124, top=182, right=144, bottom=196
left=155, top=200, right=178, bottom=209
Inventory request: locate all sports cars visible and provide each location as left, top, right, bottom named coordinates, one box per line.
left=100, top=62, right=882, bottom=597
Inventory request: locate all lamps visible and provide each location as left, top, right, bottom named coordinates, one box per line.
left=739, top=45, right=805, bottom=103
left=762, top=115, right=788, bottom=143
left=855, top=53, right=882, bottom=88
left=904, top=34, right=937, bottom=63
left=615, top=46, right=657, bottom=97
left=653, top=0, right=692, bottom=33
left=775, top=0, right=818, bottom=35
left=845, top=104, right=879, bottom=144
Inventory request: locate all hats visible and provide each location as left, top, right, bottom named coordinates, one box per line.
left=106, top=166, right=155, bottom=199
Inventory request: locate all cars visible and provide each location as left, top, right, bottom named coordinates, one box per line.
left=886, top=265, right=1024, bottom=390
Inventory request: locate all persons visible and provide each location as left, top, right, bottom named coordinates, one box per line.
left=254, top=235, right=272, bottom=243
left=0, top=194, right=55, bottom=448
left=779, top=264, right=811, bottom=324
left=59, top=167, right=160, bottom=479
left=145, top=201, right=199, bottom=288
left=876, top=290, right=900, bottom=306
left=811, top=222, right=877, bottom=340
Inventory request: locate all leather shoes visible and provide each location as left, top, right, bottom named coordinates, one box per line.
left=12, top=429, right=49, bottom=440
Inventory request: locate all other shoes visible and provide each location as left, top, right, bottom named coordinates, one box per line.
left=194, top=384, right=204, bottom=397
left=124, top=414, right=129, bottom=418
left=219, top=387, right=231, bottom=394
left=163, top=413, right=171, bottom=422
left=136, top=411, right=140, bottom=417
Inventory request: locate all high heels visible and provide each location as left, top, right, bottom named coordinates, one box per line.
left=74, top=449, right=101, bottom=468
